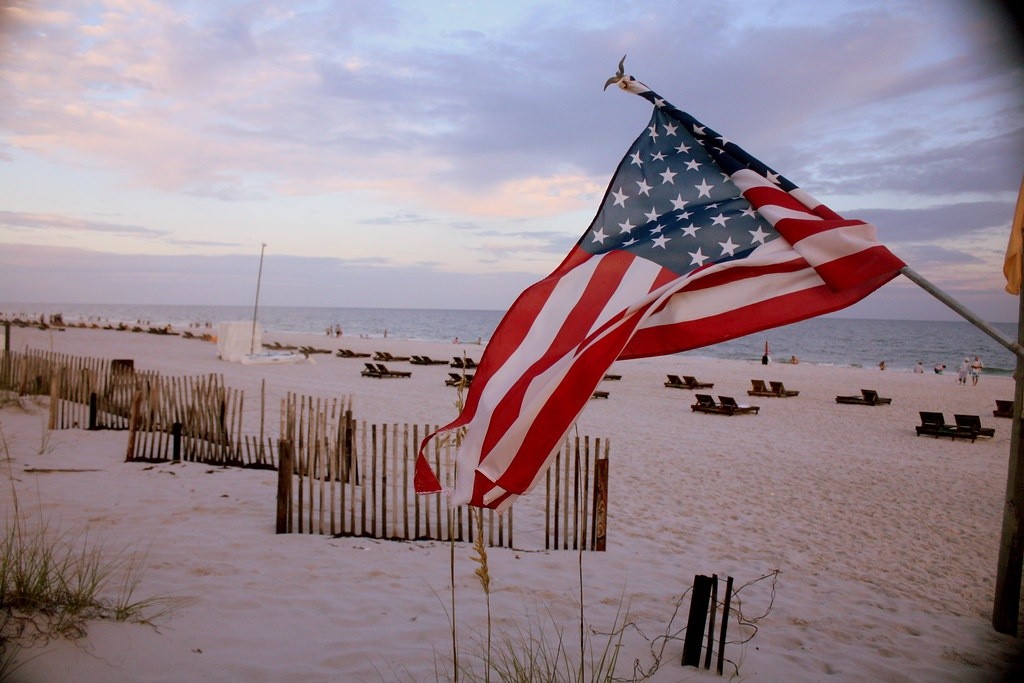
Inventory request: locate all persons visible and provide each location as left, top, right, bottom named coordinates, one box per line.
left=189, top=319, right=213, bottom=329
left=957, top=357, right=971, bottom=386
left=453, top=337, right=459, bottom=344
left=384, top=329, right=387, bottom=338
left=971, top=355, right=984, bottom=386
left=326, top=324, right=340, bottom=338
left=40, top=314, right=44, bottom=323
left=50, top=315, right=53, bottom=324
left=934, top=365, right=946, bottom=374
left=914, top=362, right=924, bottom=373
left=790, top=356, right=798, bottom=364
left=879, top=361, right=885, bottom=370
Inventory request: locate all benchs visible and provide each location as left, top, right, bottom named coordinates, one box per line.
left=0, top=313, right=1023, bottom=446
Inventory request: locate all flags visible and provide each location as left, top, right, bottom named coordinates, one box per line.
left=413, top=80, right=910, bottom=518
